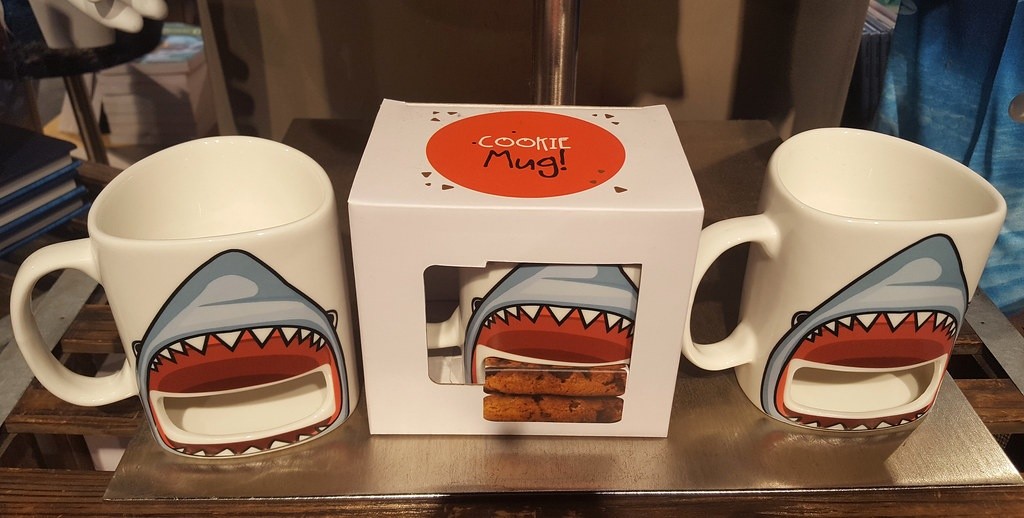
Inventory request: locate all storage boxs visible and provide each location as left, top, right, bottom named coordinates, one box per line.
left=348, top=100, right=705, bottom=437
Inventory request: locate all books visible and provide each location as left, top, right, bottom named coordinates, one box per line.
left=1, top=123, right=89, bottom=260
left=98, top=36, right=217, bottom=145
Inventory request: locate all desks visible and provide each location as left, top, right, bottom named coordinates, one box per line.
left=0, top=157, right=121, bottom=350
left=0, top=119, right=1024, bottom=518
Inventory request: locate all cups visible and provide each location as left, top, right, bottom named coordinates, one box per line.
left=425, top=261, right=642, bottom=384
left=681, top=127, right=1006, bottom=432
left=10, top=136, right=362, bottom=459
